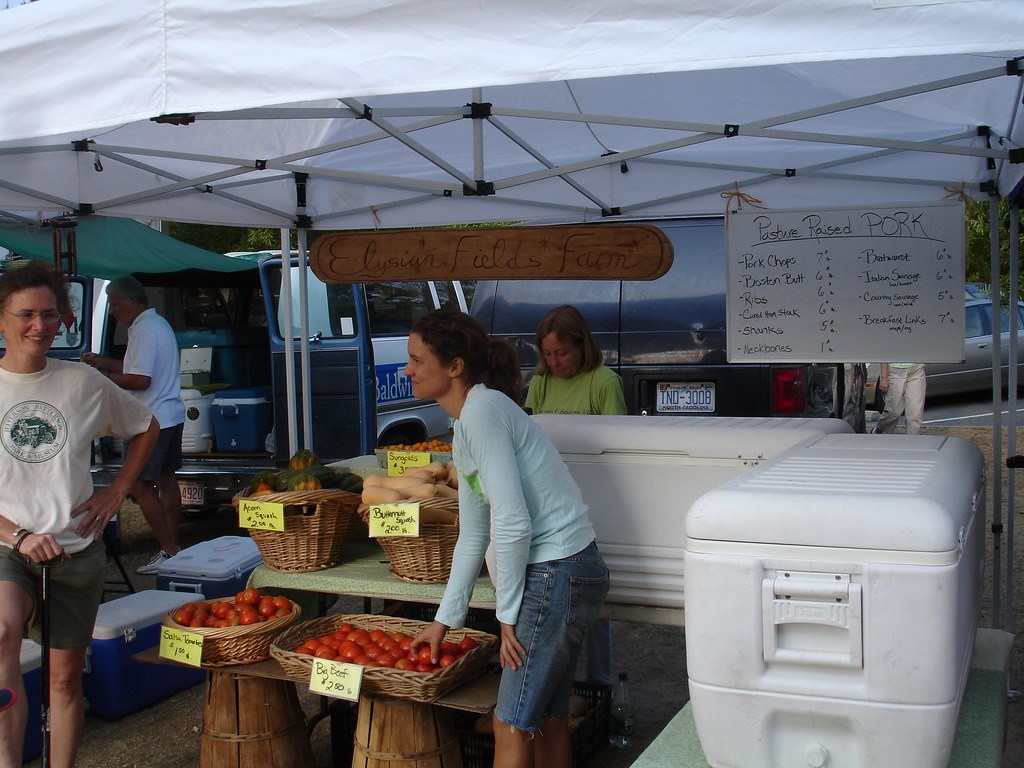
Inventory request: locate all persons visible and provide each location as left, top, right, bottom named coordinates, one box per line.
left=0, top=266, right=186, bottom=768
left=404, top=304, right=628, bottom=768
left=869, top=363, right=926, bottom=434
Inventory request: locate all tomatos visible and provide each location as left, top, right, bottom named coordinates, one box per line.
left=295, top=622, right=479, bottom=673
left=382, top=440, right=452, bottom=452
left=176, top=587, right=292, bottom=628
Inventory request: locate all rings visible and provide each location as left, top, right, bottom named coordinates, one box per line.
left=96, top=517, right=101, bottom=522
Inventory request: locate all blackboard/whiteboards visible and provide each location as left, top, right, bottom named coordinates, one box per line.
left=726, top=199, right=966, bottom=364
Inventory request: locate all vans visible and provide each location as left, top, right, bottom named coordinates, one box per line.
left=1, top=249, right=470, bottom=523
left=471, top=219, right=866, bottom=433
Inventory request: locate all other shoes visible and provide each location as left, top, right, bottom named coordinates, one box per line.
left=870, top=427, right=883, bottom=434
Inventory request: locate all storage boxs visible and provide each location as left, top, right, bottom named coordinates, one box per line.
left=527, top=414, right=856, bottom=608
left=19, top=638, right=43, bottom=764
left=329, top=698, right=358, bottom=768
left=154, top=534, right=261, bottom=598
left=178, top=372, right=273, bottom=453
left=682, top=432, right=987, bottom=768
left=454, top=679, right=614, bottom=768
left=81, top=587, right=208, bottom=721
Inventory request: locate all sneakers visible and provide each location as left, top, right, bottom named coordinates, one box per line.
left=136, top=546, right=183, bottom=575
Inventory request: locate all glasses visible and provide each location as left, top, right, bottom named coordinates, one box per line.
left=3, top=310, right=62, bottom=322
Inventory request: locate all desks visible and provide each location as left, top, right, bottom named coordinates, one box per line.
left=326, top=454, right=376, bottom=469
left=631, top=630, right=1017, bottom=768
left=245, top=551, right=682, bottom=768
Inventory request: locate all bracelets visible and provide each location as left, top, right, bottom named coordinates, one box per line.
left=107, top=372, right=110, bottom=377
left=16, top=531, right=33, bottom=551
left=12, top=528, right=27, bottom=550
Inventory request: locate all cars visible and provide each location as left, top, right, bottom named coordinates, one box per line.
left=866, top=283, right=1024, bottom=413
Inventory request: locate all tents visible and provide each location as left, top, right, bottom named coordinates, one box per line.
left=0, top=0, right=1024, bottom=702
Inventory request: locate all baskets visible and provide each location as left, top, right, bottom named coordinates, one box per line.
left=455, top=679, right=613, bottom=768
left=270, top=614, right=497, bottom=703
left=163, top=597, right=302, bottom=667
left=357, top=497, right=459, bottom=585
left=231, top=470, right=361, bottom=573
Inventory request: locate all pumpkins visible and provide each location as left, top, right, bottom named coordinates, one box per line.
left=362, top=459, right=460, bottom=524
left=242, top=449, right=363, bottom=496
